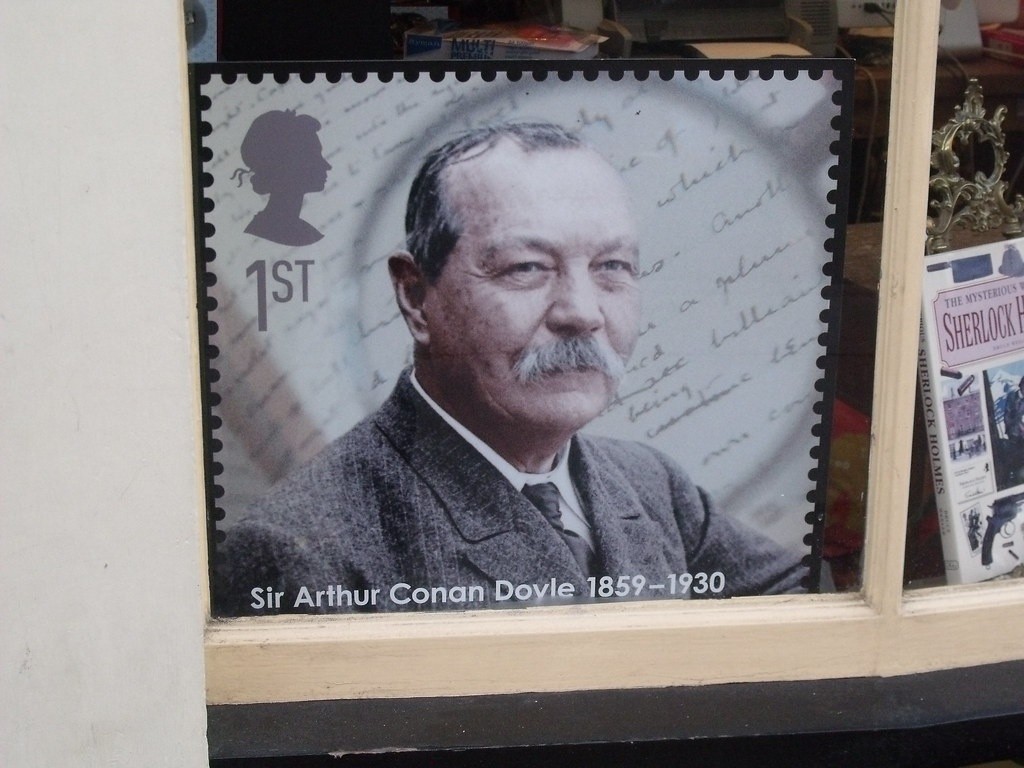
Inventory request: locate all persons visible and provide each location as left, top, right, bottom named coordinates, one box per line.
left=220, top=122, right=811, bottom=615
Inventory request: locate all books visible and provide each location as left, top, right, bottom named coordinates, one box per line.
left=918, top=236, right=1024, bottom=585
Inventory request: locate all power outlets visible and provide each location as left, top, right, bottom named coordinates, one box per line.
left=849, top=0, right=896, bottom=15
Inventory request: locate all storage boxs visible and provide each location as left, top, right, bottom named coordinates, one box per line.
left=684, top=40, right=811, bottom=60
left=979, top=23, right=1024, bottom=67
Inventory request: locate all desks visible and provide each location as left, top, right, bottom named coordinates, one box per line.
left=854, top=47, right=1024, bottom=221
left=841, top=222, right=1008, bottom=299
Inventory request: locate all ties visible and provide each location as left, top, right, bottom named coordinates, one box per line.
left=523, top=483, right=603, bottom=593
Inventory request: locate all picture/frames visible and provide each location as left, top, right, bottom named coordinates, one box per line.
left=188, top=57, right=857, bottom=620
